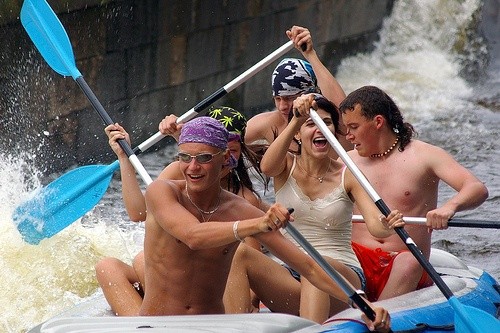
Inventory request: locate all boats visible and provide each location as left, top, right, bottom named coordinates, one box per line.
left=22, top=246, right=500, bottom=333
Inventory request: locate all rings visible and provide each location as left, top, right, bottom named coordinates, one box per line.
left=382, top=320, right=385, bottom=323
left=275, top=219, right=279, bottom=224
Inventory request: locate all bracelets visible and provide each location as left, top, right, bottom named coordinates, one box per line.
left=356, top=289, right=367, bottom=299
left=233, top=221, right=241, bottom=240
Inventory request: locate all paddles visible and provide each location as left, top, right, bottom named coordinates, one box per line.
left=308, top=104, right=500, bottom=333
left=10, top=26, right=308, bottom=247
left=351, top=214, right=500, bottom=230
left=285, top=222, right=394, bottom=333
left=20, top=0, right=154, bottom=187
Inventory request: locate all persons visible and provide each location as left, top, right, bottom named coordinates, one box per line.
left=222, top=93, right=406, bottom=324
left=139, top=116, right=391, bottom=332
left=96, top=106, right=265, bottom=317
left=337, top=86, right=488, bottom=301
left=158, top=25, right=347, bottom=265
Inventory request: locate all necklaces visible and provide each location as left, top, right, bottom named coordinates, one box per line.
left=369, top=134, right=400, bottom=158
left=298, top=155, right=331, bottom=183
left=186, top=183, right=223, bottom=222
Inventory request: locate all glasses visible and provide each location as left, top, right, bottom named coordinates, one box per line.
left=178, top=150, right=222, bottom=163
